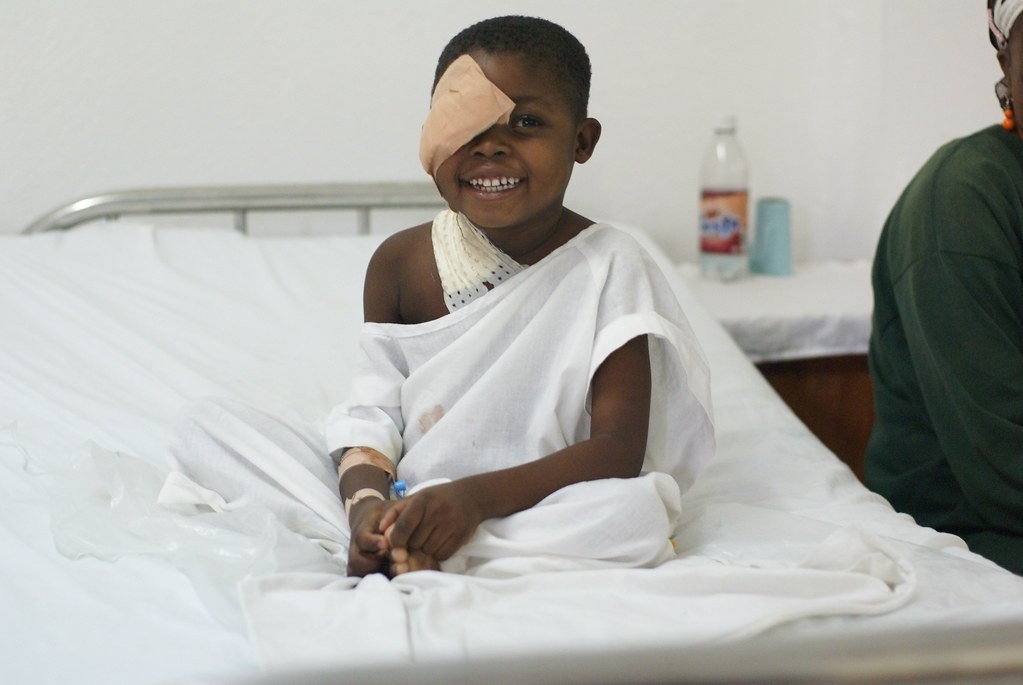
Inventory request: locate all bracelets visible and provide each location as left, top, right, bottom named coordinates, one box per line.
left=344, top=487, right=386, bottom=528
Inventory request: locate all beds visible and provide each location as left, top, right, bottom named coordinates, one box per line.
left=0, top=182, right=1023, bottom=684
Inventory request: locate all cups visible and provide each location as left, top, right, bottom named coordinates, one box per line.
left=752, top=197, right=793, bottom=277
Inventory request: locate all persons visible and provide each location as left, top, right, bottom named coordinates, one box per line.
left=337, top=16, right=653, bottom=572
left=861, top=0, right=1023, bottom=581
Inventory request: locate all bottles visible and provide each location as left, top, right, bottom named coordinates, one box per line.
left=696, top=121, right=748, bottom=280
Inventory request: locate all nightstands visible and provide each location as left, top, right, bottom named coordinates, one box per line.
left=679, top=260, right=872, bottom=483
